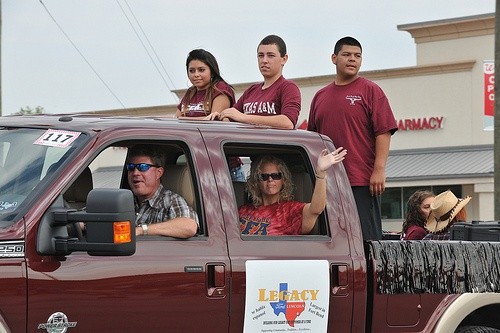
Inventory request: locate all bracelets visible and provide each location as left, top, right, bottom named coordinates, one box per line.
left=316, top=175, right=326, bottom=180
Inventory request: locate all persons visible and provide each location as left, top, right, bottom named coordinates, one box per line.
left=204, top=34, right=302, bottom=130
left=80, top=146, right=200, bottom=238
left=308, top=36, right=398, bottom=242
left=239, top=146, right=347, bottom=236
left=175, top=49, right=245, bottom=181
left=422, top=191, right=471, bottom=241
left=402, top=190, right=437, bottom=241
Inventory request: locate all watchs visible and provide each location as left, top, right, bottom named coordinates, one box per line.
left=138, top=223, right=148, bottom=236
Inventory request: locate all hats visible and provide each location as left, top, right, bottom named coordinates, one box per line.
left=424, top=190, right=473, bottom=234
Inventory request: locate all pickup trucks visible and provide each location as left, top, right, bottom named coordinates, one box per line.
left=0, top=115, right=500, bottom=332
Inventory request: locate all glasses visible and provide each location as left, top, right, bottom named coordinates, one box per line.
left=126, top=163, right=159, bottom=172
left=257, top=172, right=283, bottom=181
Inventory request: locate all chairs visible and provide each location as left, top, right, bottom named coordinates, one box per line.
left=44, top=163, right=323, bottom=236
